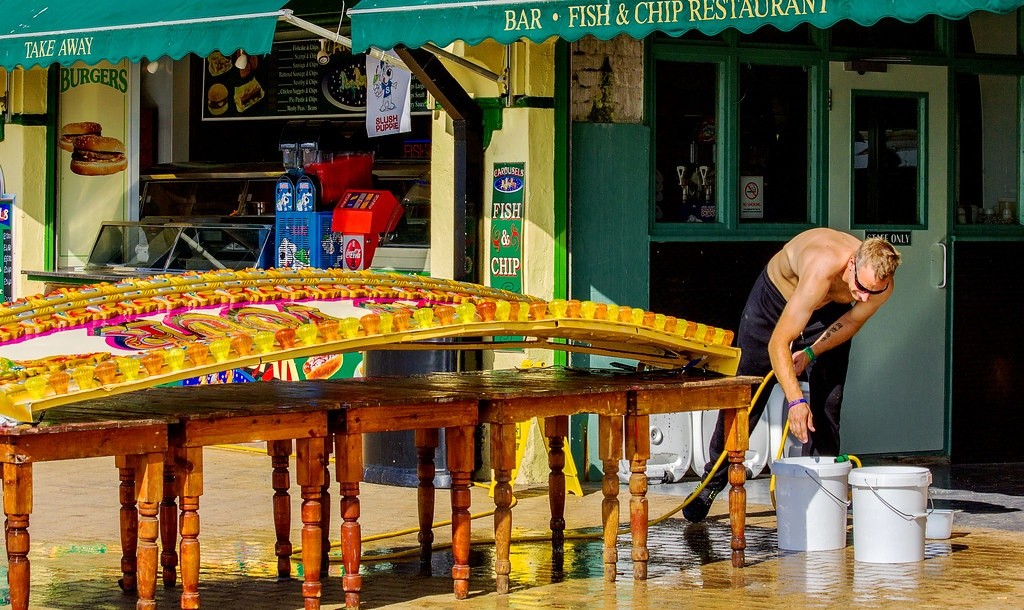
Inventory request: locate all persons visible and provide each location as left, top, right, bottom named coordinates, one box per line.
left=682, top=227, right=902, bottom=523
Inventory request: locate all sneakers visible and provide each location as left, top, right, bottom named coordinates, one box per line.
left=683, top=482, right=718, bottom=523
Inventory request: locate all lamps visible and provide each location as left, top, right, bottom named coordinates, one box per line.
left=146, top=61, right=159, bottom=74
left=317, top=39, right=330, bottom=65
left=235, top=49, right=247, bottom=70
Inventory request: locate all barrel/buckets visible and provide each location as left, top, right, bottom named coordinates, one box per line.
left=848, top=465, right=933, bottom=564
left=769, top=455, right=852, bottom=552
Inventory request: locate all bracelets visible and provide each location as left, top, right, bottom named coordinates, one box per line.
left=802, top=347, right=815, bottom=363
left=786, top=398, right=807, bottom=410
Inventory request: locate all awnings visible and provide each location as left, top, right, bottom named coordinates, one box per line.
left=0, top=0, right=1024, bottom=74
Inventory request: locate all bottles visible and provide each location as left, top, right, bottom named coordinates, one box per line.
left=350, top=239, right=356, bottom=268
left=956, top=202, right=1012, bottom=224
left=670, top=139, right=716, bottom=223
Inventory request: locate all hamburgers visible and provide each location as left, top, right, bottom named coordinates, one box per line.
left=208, top=84, right=228, bottom=115
left=61, top=122, right=128, bottom=176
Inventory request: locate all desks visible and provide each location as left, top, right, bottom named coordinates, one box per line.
left=0, top=366, right=764, bottom=610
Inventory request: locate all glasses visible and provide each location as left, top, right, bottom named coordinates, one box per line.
left=854, top=261, right=889, bottom=294
